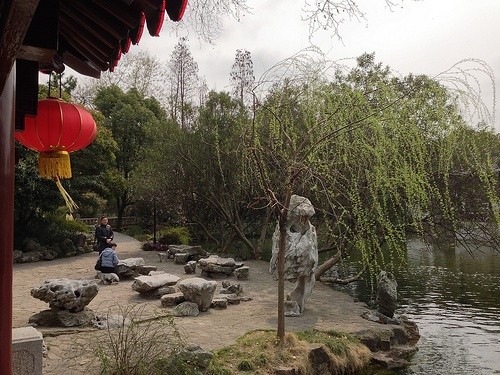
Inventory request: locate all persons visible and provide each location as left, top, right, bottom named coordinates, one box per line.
left=96, top=240, right=120, bottom=275
left=94, top=215, right=115, bottom=256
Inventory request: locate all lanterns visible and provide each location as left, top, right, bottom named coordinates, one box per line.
left=13, top=95, right=97, bottom=212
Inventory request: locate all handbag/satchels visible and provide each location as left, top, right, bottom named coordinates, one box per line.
left=94, top=260, right=102, bottom=270
